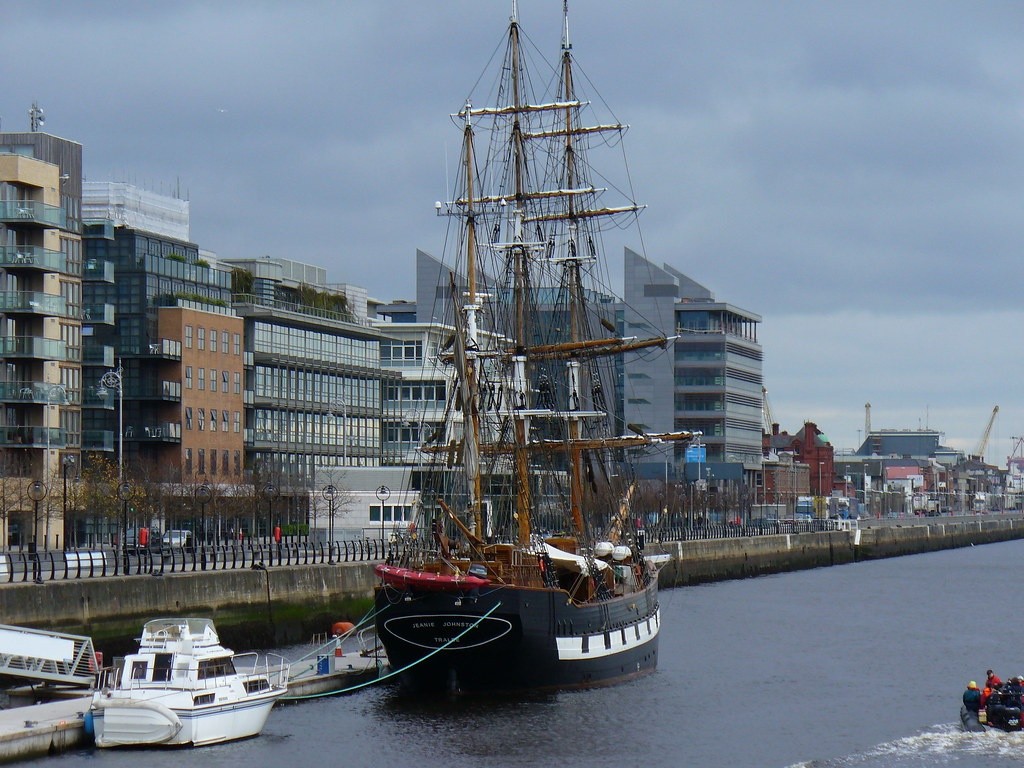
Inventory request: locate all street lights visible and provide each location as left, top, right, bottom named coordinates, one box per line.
left=327, top=398, right=434, bottom=466
left=793, top=461, right=869, bottom=519
left=27, top=481, right=49, bottom=581
left=195, top=484, right=391, bottom=571
left=96, top=358, right=126, bottom=557
left=117, top=483, right=135, bottom=576
left=45, top=385, right=66, bottom=551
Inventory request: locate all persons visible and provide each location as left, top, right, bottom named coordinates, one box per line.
left=963, top=670, right=1024, bottom=730
left=198, top=528, right=214, bottom=546
left=224, top=532, right=229, bottom=551
left=14, top=424, right=22, bottom=444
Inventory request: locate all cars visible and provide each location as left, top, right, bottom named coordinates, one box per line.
left=161, top=529, right=200, bottom=556
left=122, top=528, right=163, bottom=555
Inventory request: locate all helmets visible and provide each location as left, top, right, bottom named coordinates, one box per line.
left=967, top=681, right=976, bottom=689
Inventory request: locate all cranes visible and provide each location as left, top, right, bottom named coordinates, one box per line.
left=975, top=405, right=1001, bottom=460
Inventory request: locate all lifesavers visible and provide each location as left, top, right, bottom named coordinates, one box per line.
left=88, top=652, right=103, bottom=674
left=139, top=528, right=149, bottom=544
left=275, top=527, right=280, bottom=541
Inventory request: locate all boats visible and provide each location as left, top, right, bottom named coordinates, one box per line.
left=248, top=648, right=390, bottom=703
left=958, top=704, right=1023, bottom=733
left=374, top=1, right=705, bottom=697
left=0, top=623, right=102, bottom=762
left=92, top=616, right=292, bottom=750
